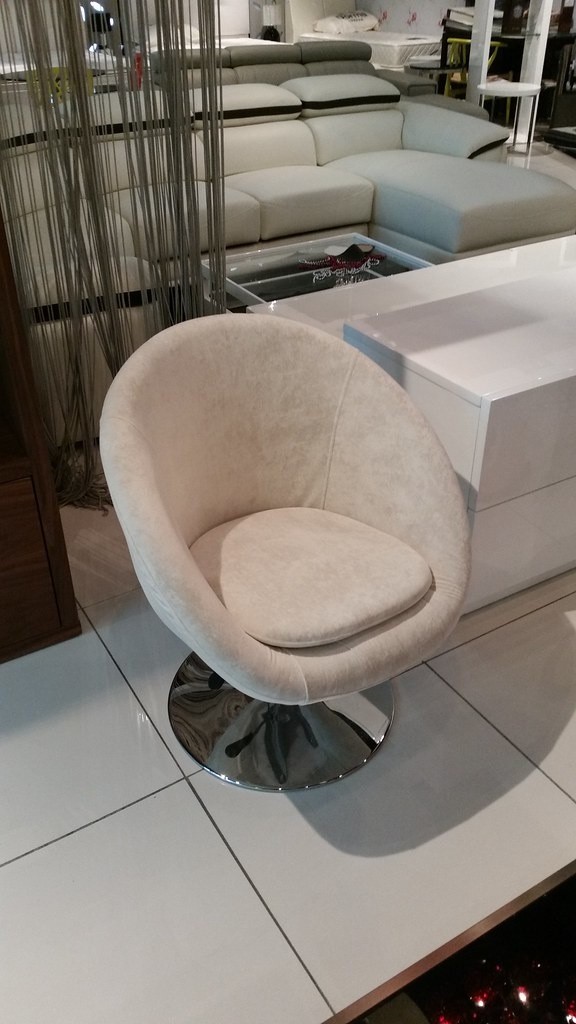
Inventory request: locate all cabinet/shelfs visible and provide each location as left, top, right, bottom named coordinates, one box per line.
left=1, top=213, right=82, bottom=666
left=247, top=234, right=575, bottom=618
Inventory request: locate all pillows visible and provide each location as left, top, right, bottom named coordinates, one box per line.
left=313, top=10, right=382, bottom=35
left=146, top=21, right=199, bottom=48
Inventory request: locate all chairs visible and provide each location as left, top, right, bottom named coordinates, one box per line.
left=444, top=38, right=513, bottom=123
left=98, top=313, right=471, bottom=791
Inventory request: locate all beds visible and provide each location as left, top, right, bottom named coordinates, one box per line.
left=283, top=0, right=461, bottom=72
left=128, top=1, right=294, bottom=61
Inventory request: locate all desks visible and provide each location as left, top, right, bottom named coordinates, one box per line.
left=200, top=233, right=435, bottom=315
left=476, top=82, right=541, bottom=149
left=439, top=32, right=576, bottom=128
left=0, top=67, right=105, bottom=99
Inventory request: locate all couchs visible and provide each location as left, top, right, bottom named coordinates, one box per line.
left=0, top=41, right=576, bottom=450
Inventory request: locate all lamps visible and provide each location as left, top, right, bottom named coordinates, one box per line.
left=262, top=5, right=283, bottom=42
left=92, top=13, right=114, bottom=48
